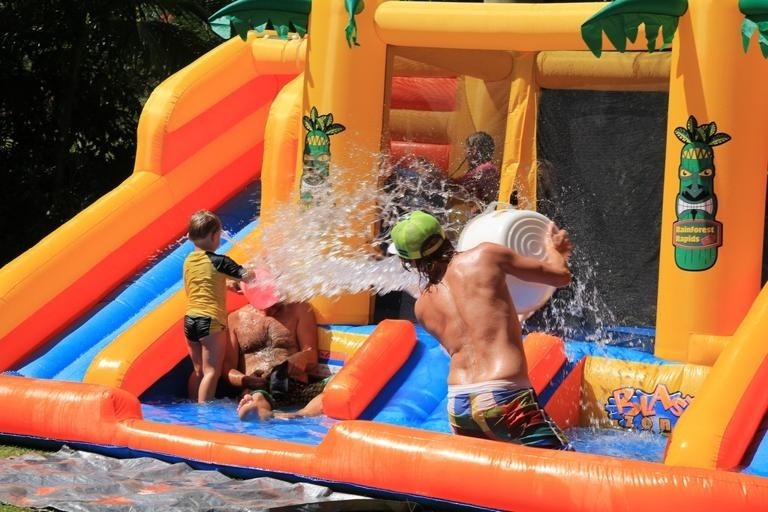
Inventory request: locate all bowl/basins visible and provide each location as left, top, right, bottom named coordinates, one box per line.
left=241, top=268, right=279, bottom=312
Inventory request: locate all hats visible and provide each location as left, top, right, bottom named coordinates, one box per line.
left=390, top=210, right=446, bottom=260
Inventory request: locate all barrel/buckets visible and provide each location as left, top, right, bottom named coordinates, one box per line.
left=239, top=265, right=282, bottom=311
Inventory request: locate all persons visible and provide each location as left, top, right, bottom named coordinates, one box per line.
left=223, top=302, right=330, bottom=420
left=183, top=210, right=256, bottom=404
left=445, top=133, right=497, bottom=213
left=386, top=210, right=574, bottom=452
